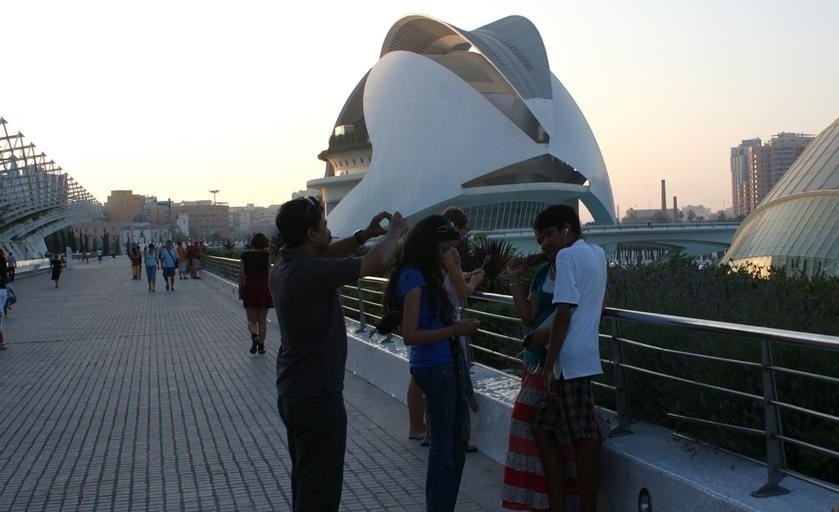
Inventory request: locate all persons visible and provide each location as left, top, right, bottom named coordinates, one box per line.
left=237, top=232, right=274, bottom=354
left=48, top=238, right=206, bottom=291
left=6, top=252, right=17, bottom=280
left=0, top=248, right=9, bottom=351
left=267, top=194, right=408, bottom=511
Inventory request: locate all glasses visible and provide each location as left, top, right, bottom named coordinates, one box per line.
left=301, top=193, right=317, bottom=218
left=431, top=219, right=456, bottom=235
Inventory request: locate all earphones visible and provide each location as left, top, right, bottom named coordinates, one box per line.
left=565, top=228, right=568, bottom=235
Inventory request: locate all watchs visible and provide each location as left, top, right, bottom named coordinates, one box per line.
left=354, top=228, right=365, bottom=246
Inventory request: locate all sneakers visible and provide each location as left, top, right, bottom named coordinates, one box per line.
left=248, top=332, right=267, bottom=355
left=406, top=432, right=481, bottom=453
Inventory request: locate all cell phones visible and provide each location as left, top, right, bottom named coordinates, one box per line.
left=465, top=318, right=481, bottom=326
